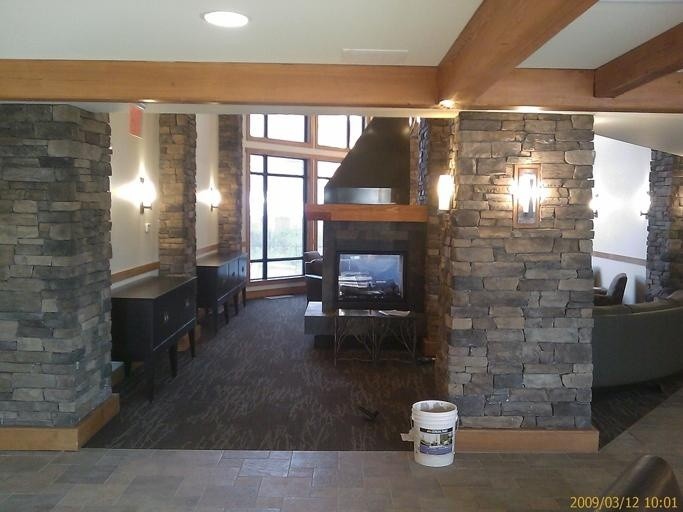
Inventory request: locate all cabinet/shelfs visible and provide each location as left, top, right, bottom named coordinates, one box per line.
left=112, top=275, right=198, bottom=402
left=195, top=249, right=251, bottom=336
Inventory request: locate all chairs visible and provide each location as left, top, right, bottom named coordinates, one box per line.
left=589, top=453, right=682, bottom=512
left=304, top=258, right=321, bottom=304
left=593, top=272, right=628, bottom=306
left=301, top=250, right=322, bottom=276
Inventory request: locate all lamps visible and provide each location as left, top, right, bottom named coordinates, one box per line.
left=210, top=184, right=223, bottom=213
left=435, top=174, right=454, bottom=211
left=137, top=174, right=155, bottom=214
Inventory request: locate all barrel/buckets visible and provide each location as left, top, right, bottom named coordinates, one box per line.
left=410, top=399, right=459, bottom=468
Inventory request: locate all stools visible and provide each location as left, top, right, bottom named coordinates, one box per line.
left=330, top=307, right=372, bottom=368
left=372, top=308, right=418, bottom=369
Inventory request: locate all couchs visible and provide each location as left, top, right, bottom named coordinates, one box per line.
left=592, top=290, right=682, bottom=389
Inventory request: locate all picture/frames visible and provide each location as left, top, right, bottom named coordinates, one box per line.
left=512, top=163, right=541, bottom=228
